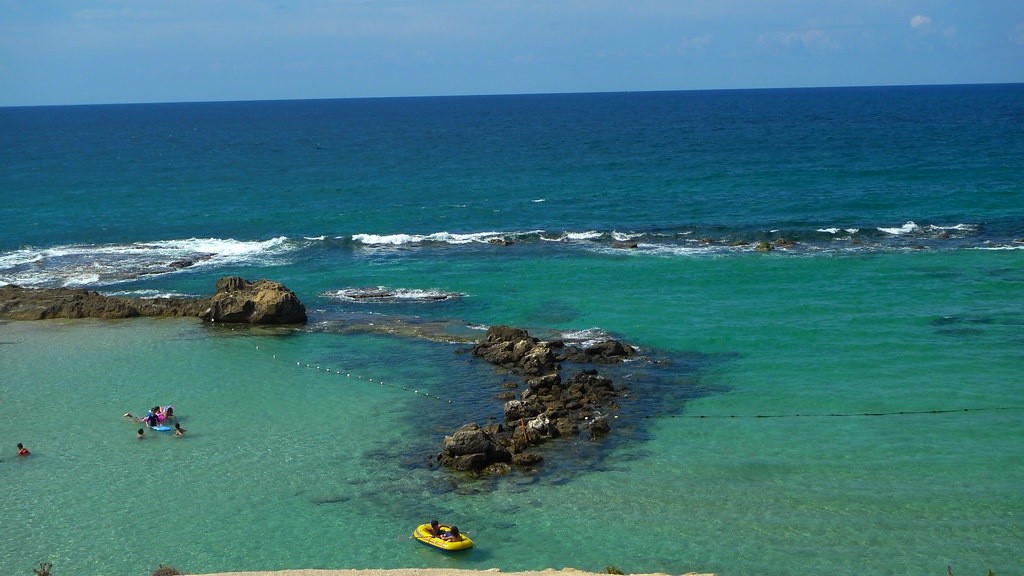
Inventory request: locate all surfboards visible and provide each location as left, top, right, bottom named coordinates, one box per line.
left=150, top=425, right=171, bottom=431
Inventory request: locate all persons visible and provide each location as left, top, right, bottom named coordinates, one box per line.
left=431, top=520, right=464, bottom=542
left=17, top=443, right=30, bottom=454
left=138, top=428, right=144, bottom=437
left=175, top=423, right=184, bottom=435
left=146, top=405, right=173, bottom=426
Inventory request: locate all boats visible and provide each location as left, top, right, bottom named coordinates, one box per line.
left=414, top=523, right=474, bottom=551
